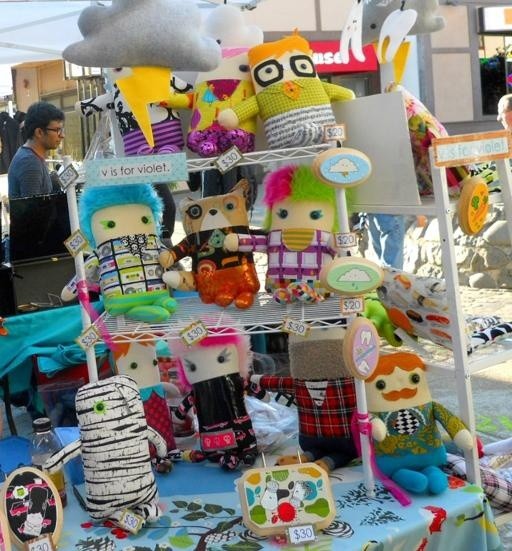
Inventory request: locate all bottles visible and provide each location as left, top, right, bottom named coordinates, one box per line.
left=30, top=417, right=67, bottom=508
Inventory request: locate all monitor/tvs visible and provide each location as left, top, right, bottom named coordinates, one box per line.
left=9, top=192, right=71, bottom=265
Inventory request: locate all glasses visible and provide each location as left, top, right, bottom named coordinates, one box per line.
left=47, top=127, right=62, bottom=134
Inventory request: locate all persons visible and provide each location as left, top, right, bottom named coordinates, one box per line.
left=497, top=94, right=512, bottom=128
left=7, top=102, right=66, bottom=197
left=358, top=213, right=405, bottom=272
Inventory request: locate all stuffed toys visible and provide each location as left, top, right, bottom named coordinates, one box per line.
left=171, top=325, right=271, bottom=472
left=110, top=324, right=185, bottom=460
left=223, top=163, right=339, bottom=306
left=157, top=187, right=268, bottom=309
left=42, top=374, right=167, bottom=523
left=61, top=0, right=356, bottom=157
left=60, top=0, right=357, bottom=157
left=59, top=181, right=185, bottom=324
left=364, top=351, right=474, bottom=493
left=249, top=327, right=361, bottom=474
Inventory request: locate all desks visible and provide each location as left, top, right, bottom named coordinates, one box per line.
left=0, top=417, right=502, bottom=551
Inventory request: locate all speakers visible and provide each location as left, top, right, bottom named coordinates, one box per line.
left=481, top=63, right=505, bottom=114
